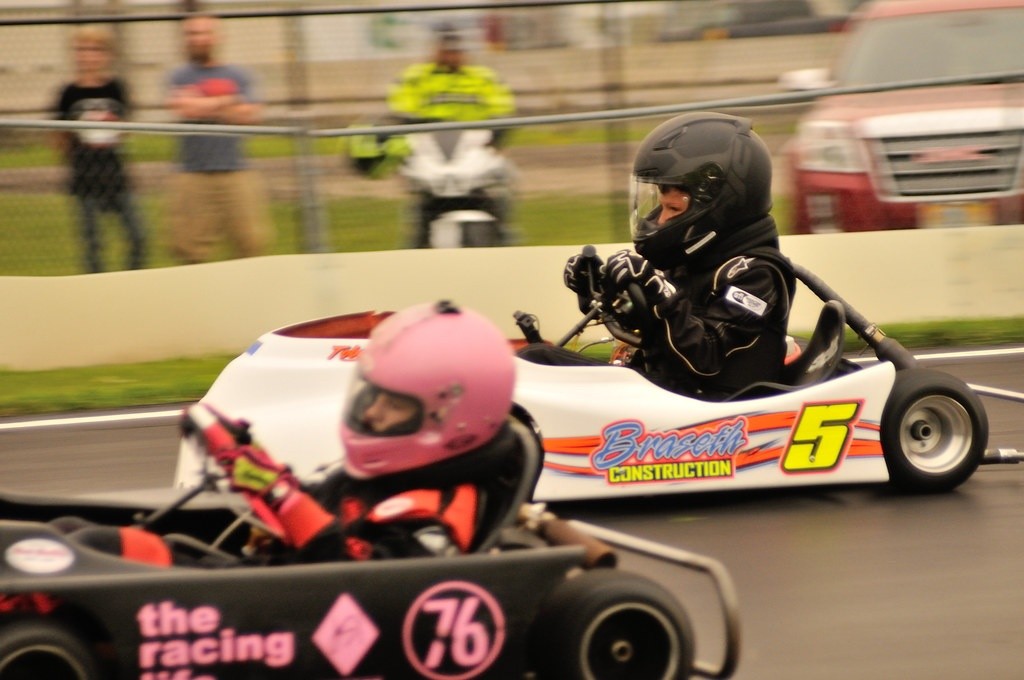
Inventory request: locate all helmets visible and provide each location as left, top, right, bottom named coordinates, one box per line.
left=629, top=112, right=773, bottom=269
left=339, top=299, right=516, bottom=476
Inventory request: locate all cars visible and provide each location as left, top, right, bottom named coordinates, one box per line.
left=774, top=0, right=1023, bottom=233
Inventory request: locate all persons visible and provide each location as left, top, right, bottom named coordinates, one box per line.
left=558, top=113, right=796, bottom=403
left=54, top=11, right=517, bottom=276
left=177, top=300, right=518, bottom=568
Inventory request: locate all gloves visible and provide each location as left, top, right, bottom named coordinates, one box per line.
left=599, top=249, right=680, bottom=319
left=564, top=245, right=606, bottom=320
left=216, top=446, right=301, bottom=515
left=180, top=403, right=252, bottom=445
